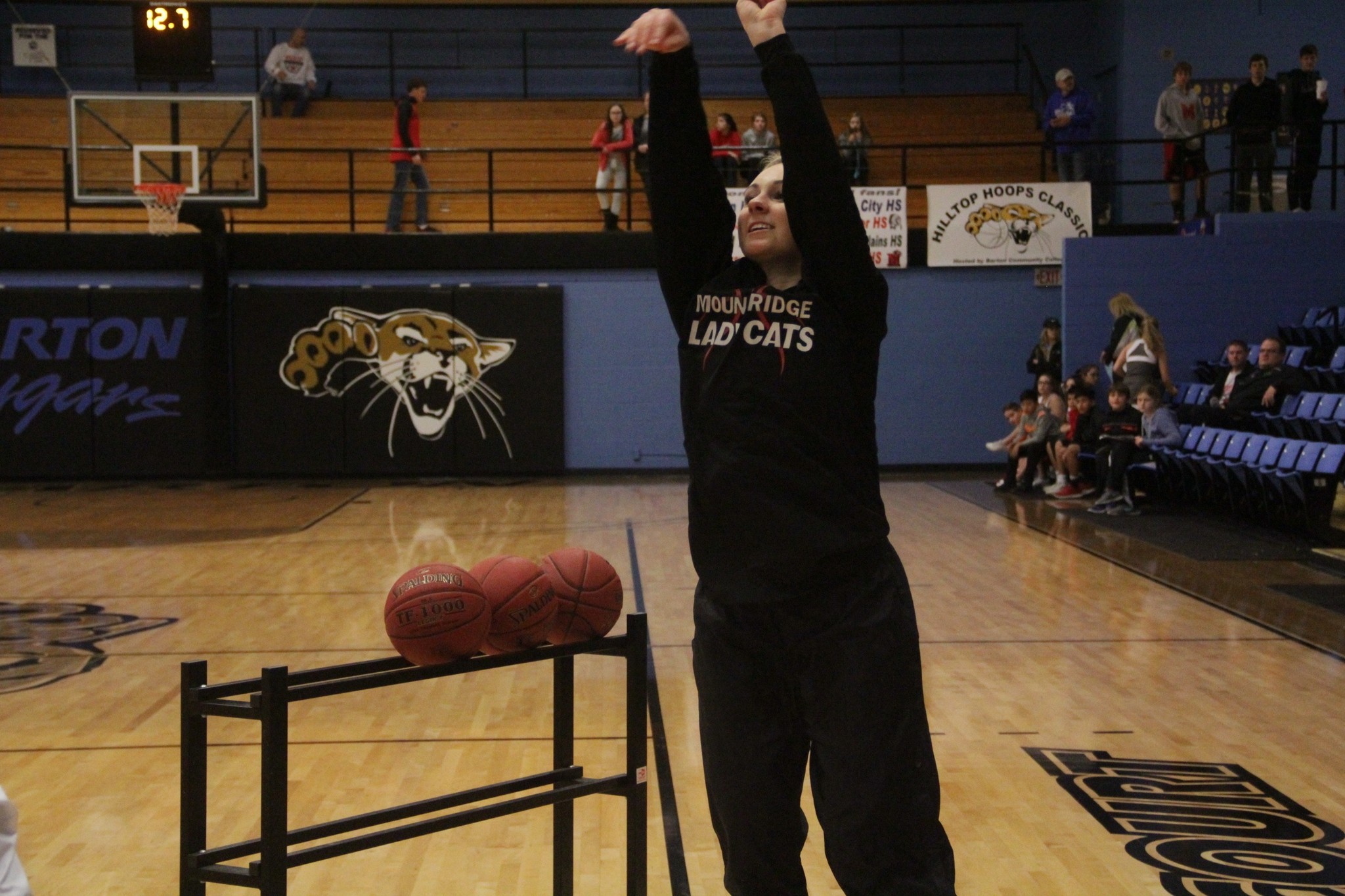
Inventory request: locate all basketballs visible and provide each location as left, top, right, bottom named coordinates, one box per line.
left=467, top=555, right=558, bottom=655
left=542, top=549, right=623, bottom=646
left=384, top=563, right=492, bottom=666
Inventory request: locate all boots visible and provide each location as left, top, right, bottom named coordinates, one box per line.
left=603, top=209, right=621, bottom=232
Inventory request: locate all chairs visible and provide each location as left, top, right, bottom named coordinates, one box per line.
left=1079, top=305, right=1345, bottom=533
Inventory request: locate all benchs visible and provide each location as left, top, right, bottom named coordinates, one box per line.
left=0, top=96, right=1057, bottom=234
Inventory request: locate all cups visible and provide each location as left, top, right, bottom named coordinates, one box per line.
left=1316, top=80, right=1329, bottom=98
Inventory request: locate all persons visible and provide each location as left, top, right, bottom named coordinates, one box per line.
left=1203, top=337, right=1304, bottom=434
left=984, top=293, right=1182, bottom=516
left=1154, top=62, right=1212, bottom=225
left=385, top=82, right=440, bottom=232
left=1042, top=70, right=1114, bottom=223
left=1225, top=44, right=1329, bottom=213
left=613, top=0, right=958, bottom=896
left=264, top=27, right=318, bottom=117
left=592, top=92, right=874, bottom=233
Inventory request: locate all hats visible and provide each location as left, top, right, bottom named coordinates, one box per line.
left=1043, top=317, right=1061, bottom=329
left=1055, top=69, right=1075, bottom=82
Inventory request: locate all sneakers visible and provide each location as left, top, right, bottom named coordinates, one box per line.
left=994, top=475, right=1151, bottom=518
left=417, top=225, right=440, bottom=233
